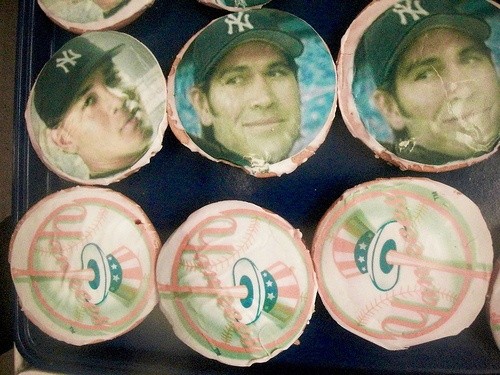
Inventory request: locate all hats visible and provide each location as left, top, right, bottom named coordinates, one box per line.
left=34, top=36, right=126, bottom=130
left=365, top=0, right=492, bottom=87
left=194, top=9, right=305, bottom=83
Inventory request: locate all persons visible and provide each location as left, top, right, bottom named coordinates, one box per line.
left=34, top=35, right=154, bottom=180
left=364, top=0, right=500, bottom=166
left=188, top=11, right=304, bottom=167
